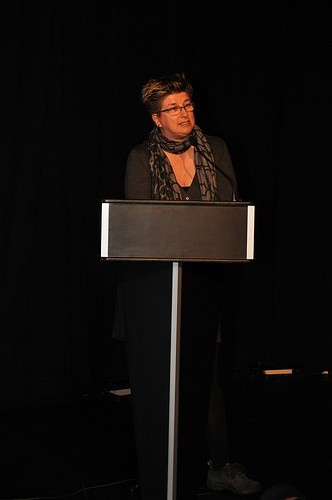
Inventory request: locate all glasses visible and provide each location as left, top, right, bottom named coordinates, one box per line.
left=157, top=102, right=194, bottom=116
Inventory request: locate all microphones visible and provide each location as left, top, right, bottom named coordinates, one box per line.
left=189, top=136, right=242, bottom=202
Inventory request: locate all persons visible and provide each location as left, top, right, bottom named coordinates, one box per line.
left=112, top=72, right=261, bottom=500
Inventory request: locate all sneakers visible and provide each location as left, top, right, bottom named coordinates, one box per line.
left=207, top=463, right=260, bottom=495
